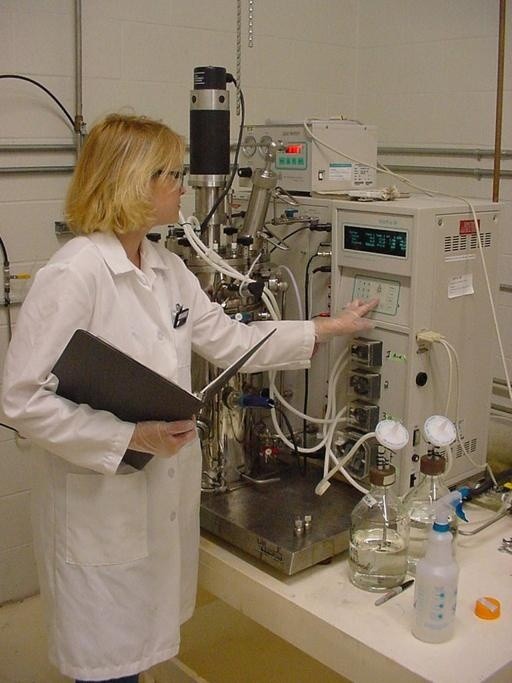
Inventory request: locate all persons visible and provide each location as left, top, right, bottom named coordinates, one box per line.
left=0, top=112, right=382, bottom=683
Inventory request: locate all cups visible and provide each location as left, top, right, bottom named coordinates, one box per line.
left=154, top=166, right=190, bottom=189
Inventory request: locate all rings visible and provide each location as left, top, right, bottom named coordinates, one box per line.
left=375, top=579, right=415, bottom=606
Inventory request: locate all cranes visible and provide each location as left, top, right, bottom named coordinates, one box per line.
left=48, top=328, right=278, bottom=469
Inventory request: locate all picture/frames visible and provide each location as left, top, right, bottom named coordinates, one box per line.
left=144, top=493, right=511, bottom=683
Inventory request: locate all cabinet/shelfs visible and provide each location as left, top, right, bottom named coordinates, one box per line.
left=348, top=454, right=458, bottom=593
